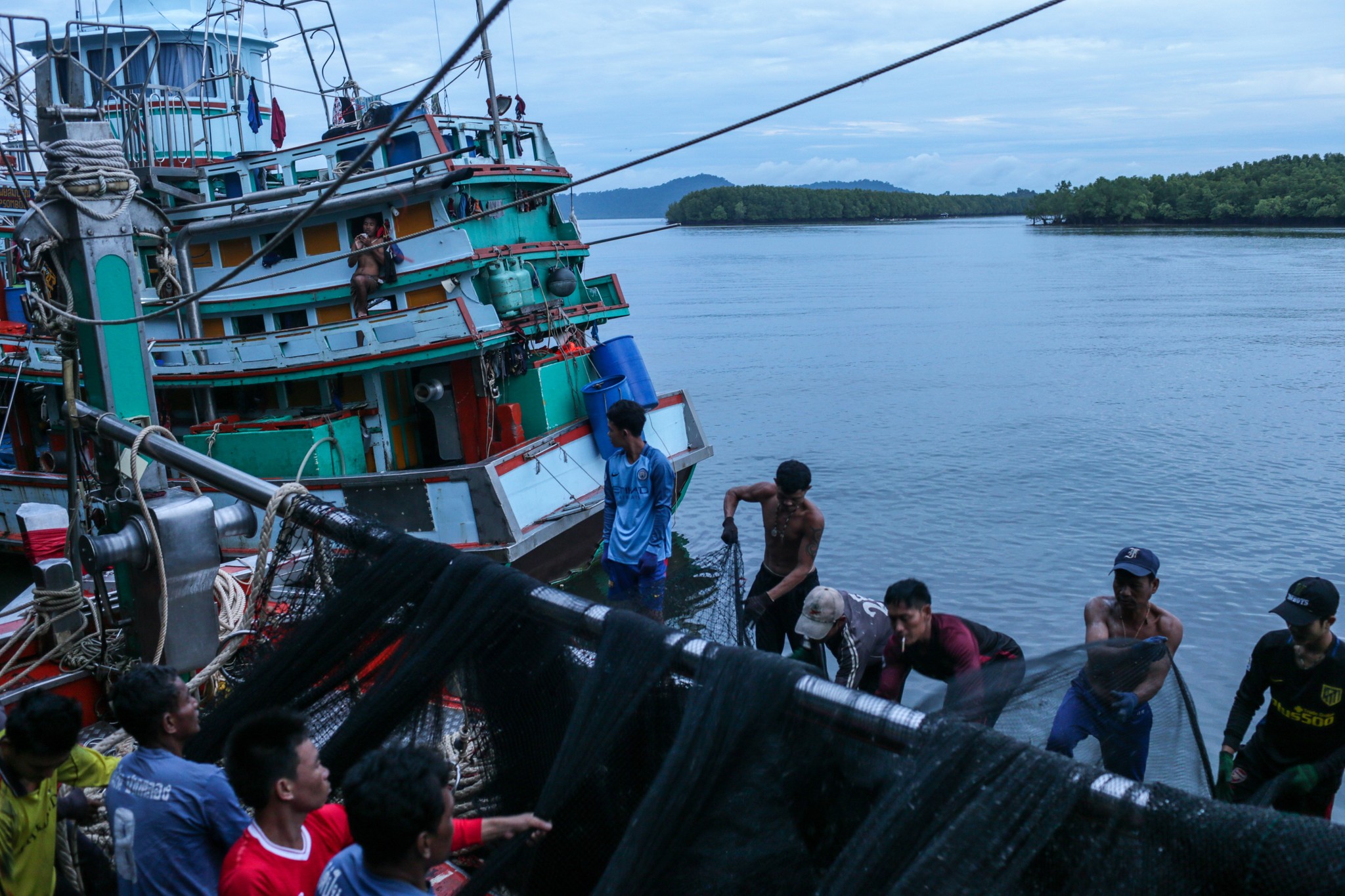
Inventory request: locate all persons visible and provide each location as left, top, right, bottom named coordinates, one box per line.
left=346, top=214, right=385, bottom=319
left=315, top=742, right=460, bottom=896
left=217, top=717, right=555, bottom=896
left=1213, top=576, right=1345, bottom=815
left=1045, top=546, right=1183, bottom=782
left=0, top=690, right=124, bottom=896
left=601, top=399, right=676, bottom=625
left=794, top=585, right=894, bottom=696
left=876, top=578, right=1026, bottom=730
left=104, top=664, right=254, bottom=896
left=721, top=460, right=830, bottom=683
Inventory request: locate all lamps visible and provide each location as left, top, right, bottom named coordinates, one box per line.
left=441, top=276, right=459, bottom=294
left=485, top=94, right=511, bottom=119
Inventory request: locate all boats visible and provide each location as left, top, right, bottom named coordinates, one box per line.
left=0, top=2, right=716, bottom=590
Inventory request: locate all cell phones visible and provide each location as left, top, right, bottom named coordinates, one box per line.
left=361, top=234, right=367, bottom=238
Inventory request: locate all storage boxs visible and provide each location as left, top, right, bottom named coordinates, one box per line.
left=0, top=320, right=26, bottom=354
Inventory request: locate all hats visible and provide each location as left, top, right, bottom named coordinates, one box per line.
left=1268, top=576, right=1340, bottom=626
left=1108, top=546, right=1160, bottom=579
left=794, top=586, right=845, bottom=641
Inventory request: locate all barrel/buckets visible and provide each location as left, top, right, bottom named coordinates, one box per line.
left=589, top=335, right=659, bottom=414
left=580, top=374, right=646, bottom=461
left=465, top=135, right=476, bottom=158
left=4, top=284, right=32, bottom=333
left=387, top=100, right=428, bottom=168
left=223, top=156, right=261, bottom=199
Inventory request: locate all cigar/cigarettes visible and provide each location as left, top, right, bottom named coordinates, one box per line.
left=902, top=637, right=905, bottom=653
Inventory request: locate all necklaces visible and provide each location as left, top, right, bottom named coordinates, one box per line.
left=1117, top=601, right=1151, bottom=646
left=1297, top=643, right=1325, bottom=669
left=771, top=501, right=802, bottom=539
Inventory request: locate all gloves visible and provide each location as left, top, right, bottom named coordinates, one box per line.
left=1273, top=765, right=1319, bottom=801
left=789, top=648, right=812, bottom=662
left=1106, top=690, right=1137, bottom=726
left=1132, top=635, right=1168, bottom=662
left=1212, top=751, right=1234, bottom=804
left=721, top=516, right=739, bottom=544
left=741, top=591, right=775, bottom=631
